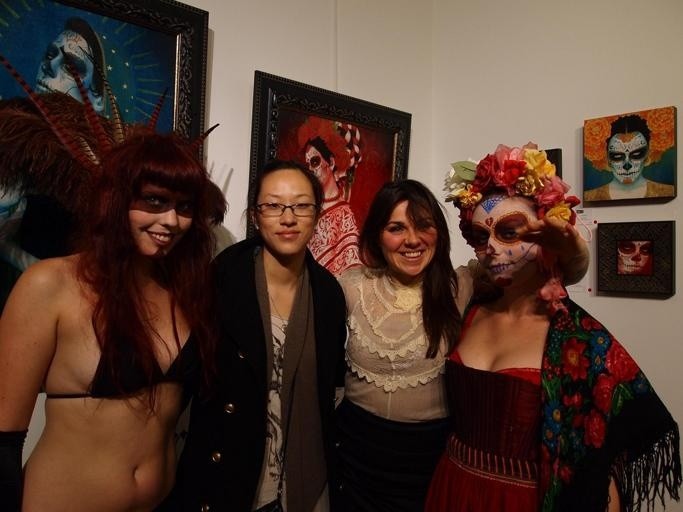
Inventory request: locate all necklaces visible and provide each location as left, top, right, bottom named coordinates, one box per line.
left=264, top=290, right=290, bottom=335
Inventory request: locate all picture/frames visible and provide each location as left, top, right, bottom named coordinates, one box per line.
left=539, top=149, right=562, bottom=179
left=0, top=0, right=210, bottom=392
left=246, top=70, right=412, bottom=279
left=597, top=221, right=676, bottom=299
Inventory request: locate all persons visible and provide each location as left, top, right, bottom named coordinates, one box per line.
left=293, top=113, right=362, bottom=277
left=169, top=159, right=348, bottom=511
left=583, top=113, right=674, bottom=202
left=418, top=140, right=682, bottom=512
left=0, top=131, right=216, bottom=510
left=0, top=15, right=107, bottom=314
left=332, top=178, right=483, bottom=512
left=616, top=238, right=651, bottom=276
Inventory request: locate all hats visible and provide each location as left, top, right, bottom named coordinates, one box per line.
left=63, top=15, right=138, bottom=124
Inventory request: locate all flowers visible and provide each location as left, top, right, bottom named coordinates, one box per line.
left=443, top=142, right=581, bottom=227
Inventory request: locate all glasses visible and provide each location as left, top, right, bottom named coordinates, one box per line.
left=250, top=200, right=320, bottom=218
